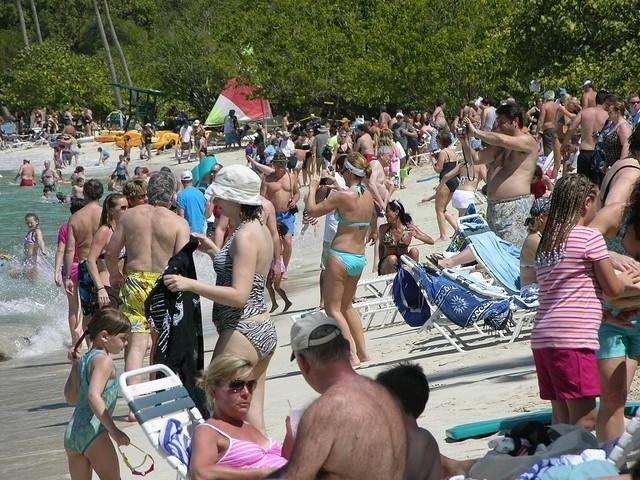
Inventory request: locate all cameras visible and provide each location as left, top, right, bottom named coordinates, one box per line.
left=456, top=122, right=468, bottom=135
left=592, top=131, right=599, bottom=138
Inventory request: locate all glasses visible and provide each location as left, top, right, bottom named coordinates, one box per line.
left=340, top=135, right=346, bottom=138
left=120, top=205, right=127, bottom=211
left=630, top=100, right=640, bottom=105
left=396, top=116, right=404, bottom=119
left=227, top=378, right=257, bottom=393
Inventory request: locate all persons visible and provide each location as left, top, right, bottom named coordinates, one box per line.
left=602, top=102, right=631, bottom=167
left=189, top=354, right=294, bottom=480
left=276, top=310, right=408, bottom=479
left=39, top=161, right=59, bottom=200
left=197, top=132, right=208, bottom=159
left=111, top=156, right=129, bottom=181
left=88, top=193, right=129, bottom=307
left=458, top=104, right=538, bottom=250
left=535, top=91, right=576, bottom=156
left=50, top=122, right=83, bottom=166
left=96, top=147, right=108, bottom=167
left=304, top=155, right=348, bottom=315
left=179, top=122, right=193, bottom=163
left=530, top=174, right=639, bottom=432
left=520, top=198, right=551, bottom=300
left=64, top=179, right=106, bottom=348
left=307, top=152, right=374, bottom=365
left=561, top=92, right=609, bottom=174
left=365, top=145, right=393, bottom=271
left=459, top=105, right=498, bottom=150
left=141, top=123, right=151, bottom=160
left=378, top=199, right=434, bottom=294
left=375, top=361, right=444, bottom=479
left=630, top=94, right=640, bottom=126
left=71, top=167, right=86, bottom=186
left=164, top=165, right=277, bottom=435
left=63, top=306, right=130, bottom=480
left=191, top=120, right=204, bottom=155
left=427, top=238, right=516, bottom=285
left=123, top=176, right=149, bottom=208
left=239, top=98, right=454, bottom=166
left=175, top=171, right=208, bottom=236
left=106, top=171, right=190, bottom=421
left=122, top=135, right=132, bottom=163
left=588, top=178, right=638, bottom=444
left=597, top=124, right=640, bottom=207
left=430, top=134, right=459, bottom=242
left=266, top=223, right=292, bottom=315
left=54, top=198, right=88, bottom=352
left=15, top=160, right=35, bottom=186
left=259, top=152, right=301, bottom=280
left=1, top=110, right=53, bottom=145
left=1, top=211, right=47, bottom=287
left=581, top=79, right=596, bottom=108
left=58, top=107, right=96, bottom=131
left=223, top=110, right=237, bottom=149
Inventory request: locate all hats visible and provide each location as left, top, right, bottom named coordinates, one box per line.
left=180, top=170, right=193, bottom=180
left=582, top=80, right=591, bottom=87
left=192, top=119, right=200, bottom=126
left=144, top=123, right=152, bottom=127
left=317, top=125, right=328, bottom=132
left=205, top=164, right=262, bottom=206
left=339, top=117, right=350, bottom=123
left=396, top=112, right=404, bottom=117
left=272, top=151, right=288, bottom=162
left=289, top=308, right=341, bottom=363
left=336, top=154, right=367, bottom=178
left=559, top=88, right=567, bottom=95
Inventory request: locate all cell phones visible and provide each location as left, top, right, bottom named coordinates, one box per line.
left=319, top=177, right=328, bottom=186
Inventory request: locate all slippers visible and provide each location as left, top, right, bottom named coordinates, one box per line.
left=431, top=253, right=439, bottom=264
left=426, top=254, right=444, bottom=270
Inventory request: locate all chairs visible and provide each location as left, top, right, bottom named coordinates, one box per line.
left=119, top=364, right=210, bottom=479
left=400, top=252, right=539, bottom=353
left=457, top=213, right=524, bottom=294
left=292, top=272, right=401, bottom=331
